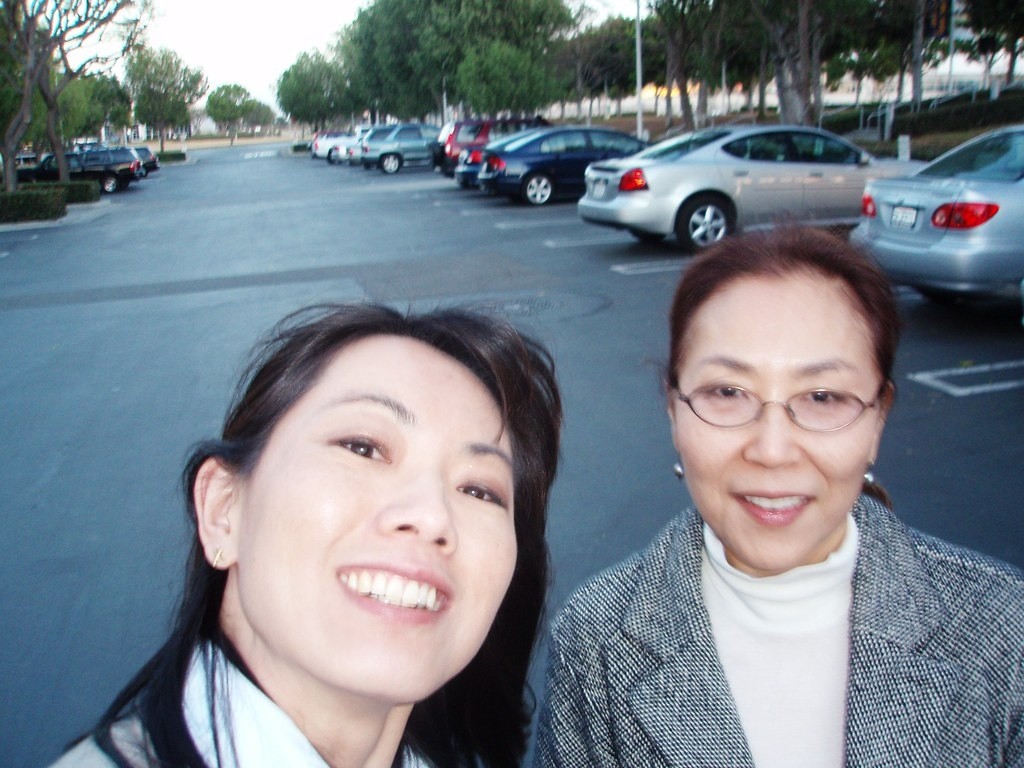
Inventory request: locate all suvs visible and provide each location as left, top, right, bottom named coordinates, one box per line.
left=430, top=117, right=551, bottom=179
left=15, top=141, right=161, bottom=194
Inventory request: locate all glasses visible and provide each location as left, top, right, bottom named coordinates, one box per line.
left=673, top=384, right=879, bottom=433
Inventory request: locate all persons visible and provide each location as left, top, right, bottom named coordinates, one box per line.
left=50, top=301, right=562, bottom=767
left=533, top=222, right=1023, bottom=767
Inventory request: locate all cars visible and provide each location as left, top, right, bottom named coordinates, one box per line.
left=576, top=120, right=930, bottom=257
left=845, top=125, right=1024, bottom=329
left=306, top=122, right=441, bottom=176
left=455, top=126, right=555, bottom=190
left=477, top=125, right=656, bottom=208
